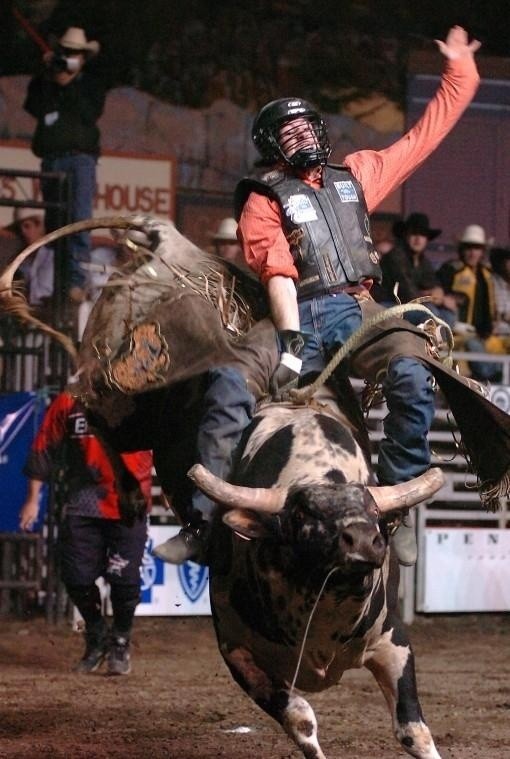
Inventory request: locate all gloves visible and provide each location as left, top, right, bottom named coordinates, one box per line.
left=268, top=328, right=310, bottom=404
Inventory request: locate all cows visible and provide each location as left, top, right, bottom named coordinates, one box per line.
left=0, top=210, right=447, bottom=757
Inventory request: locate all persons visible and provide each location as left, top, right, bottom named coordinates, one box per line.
left=18, top=342, right=153, bottom=676
left=152, top=25, right=483, bottom=569
left=25, top=25, right=109, bottom=305
left=0, top=203, right=90, bottom=391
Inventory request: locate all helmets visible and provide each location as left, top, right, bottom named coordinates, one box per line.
left=249, top=97, right=334, bottom=172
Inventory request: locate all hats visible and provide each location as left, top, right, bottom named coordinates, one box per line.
left=205, top=216, right=240, bottom=242
left=53, top=26, right=102, bottom=64
left=452, top=223, right=496, bottom=248
left=392, top=212, right=443, bottom=242
left=2, top=203, right=45, bottom=228
left=108, top=225, right=153, bottom=248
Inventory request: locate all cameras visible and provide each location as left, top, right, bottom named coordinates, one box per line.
left=50, top=57, right=80, bottom=75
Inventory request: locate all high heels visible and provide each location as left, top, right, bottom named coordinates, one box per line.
left=150, top=511, right=214, bottom=569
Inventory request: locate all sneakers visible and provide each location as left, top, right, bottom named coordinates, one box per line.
left=105, top=634, right=133, bottom=676
left=71, top=638, right=110, bottom=674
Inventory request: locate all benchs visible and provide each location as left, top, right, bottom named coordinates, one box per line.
left=73, top=295, right=506, bottom=526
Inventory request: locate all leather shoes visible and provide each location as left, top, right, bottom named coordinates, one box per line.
left=390, top=506, right=419, bottom=568
